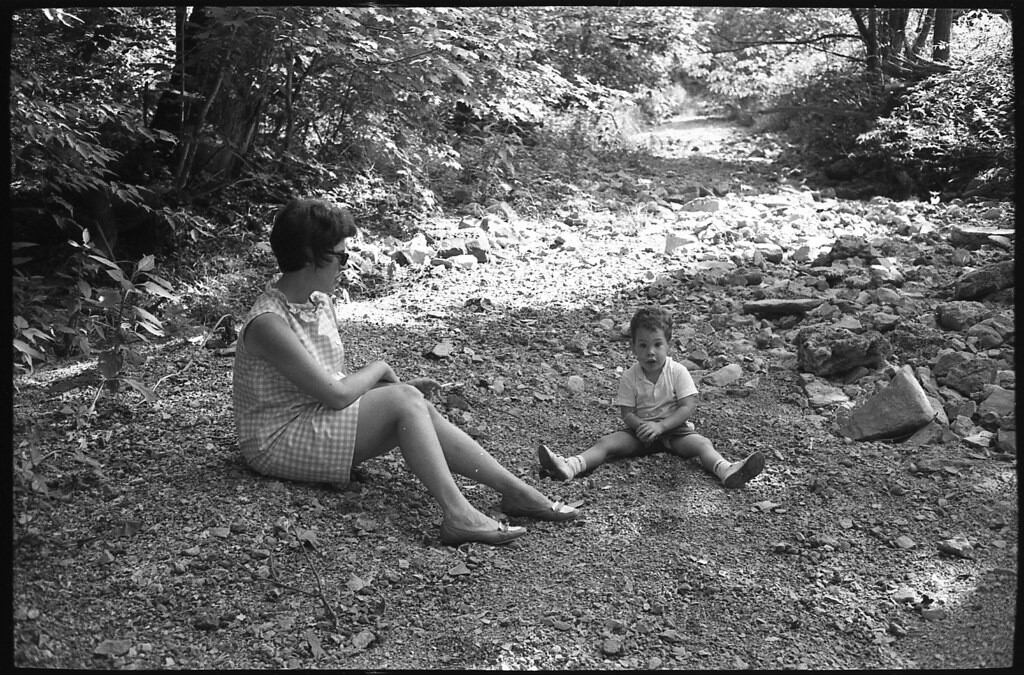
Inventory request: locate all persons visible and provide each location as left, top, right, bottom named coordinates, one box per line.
left=233, top=201, right=580, bottom=544
left=539, top=309, right=765, bottom=488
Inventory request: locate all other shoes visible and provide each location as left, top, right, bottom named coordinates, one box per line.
left=539, top=445, right=574, bottom=482
left=722, top=451, right=765, bottom=489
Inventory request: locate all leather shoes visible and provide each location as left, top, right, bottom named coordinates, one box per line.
left=501, top=496, right=581, bottom=522
left=438, top=518, right=527, bottom=548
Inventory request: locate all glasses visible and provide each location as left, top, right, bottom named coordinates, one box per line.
left=327, top=252, right=351, bottom=270
